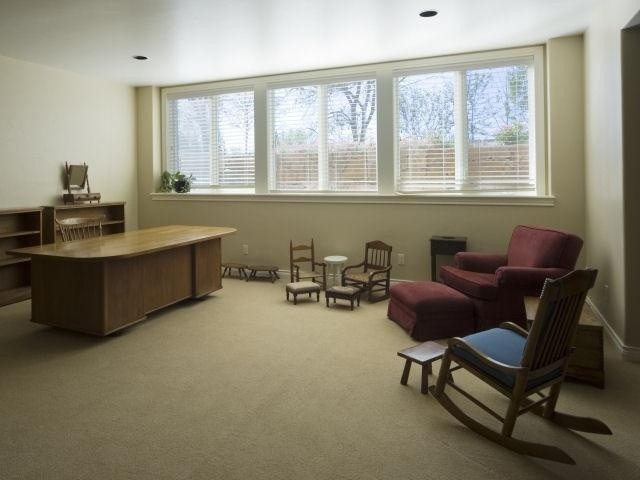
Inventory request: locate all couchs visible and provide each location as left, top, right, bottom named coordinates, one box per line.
left=386, top=225, right=584, bottom=342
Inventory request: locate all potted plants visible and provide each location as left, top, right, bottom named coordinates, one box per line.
left=156, top=170, right=172, bottom=193
left=173, top=171, right=192, bottom=193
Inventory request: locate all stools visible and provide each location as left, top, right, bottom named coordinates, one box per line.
left=397, top=340, right=454, bottom=395
left=221, top=262, right=248, bottom=280
left=246, top=264, right=280, bottom=283
left=325, top=285, right=361, bottom=311
left=324, top=255, right=348, bottom=287
left=286, top=281, right=320, bottom=305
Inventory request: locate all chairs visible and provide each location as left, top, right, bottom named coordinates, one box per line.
left=341, top=239, right=393, bottom=297
left=428, top=268, right=614, bottom=466
left=290, top=238, right=327, bottom=291
left=55, top=214, right=107, bottom=242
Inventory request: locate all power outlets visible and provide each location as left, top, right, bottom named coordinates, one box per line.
left=397, top=253, right=406, bottom=266
left=242, top=244, right=249, bottom=255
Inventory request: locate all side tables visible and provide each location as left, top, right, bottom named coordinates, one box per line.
left=523, top=296, right=605, bottom=390
left=428, top=235, right=468, bottom=282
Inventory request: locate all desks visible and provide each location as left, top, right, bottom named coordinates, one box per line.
left=5, top=224, right=238, bottom=338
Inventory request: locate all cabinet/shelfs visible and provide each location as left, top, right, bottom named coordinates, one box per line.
left=41, top=202, right=127, bottom=245
left=0, top=207, right=43, bottom=308
